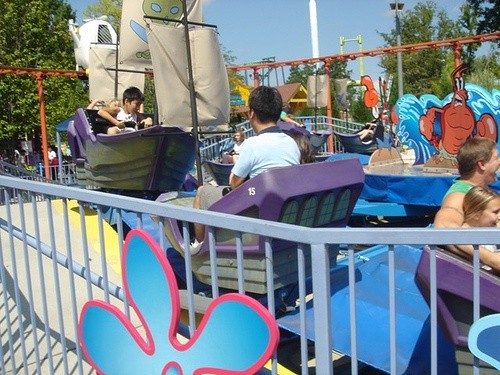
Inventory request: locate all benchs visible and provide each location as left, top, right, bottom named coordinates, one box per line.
left=73, top=109, right=147, bottom=137
left=414, top=223, right=500, bottom=375
left=149, top=159, right=365, bottom=294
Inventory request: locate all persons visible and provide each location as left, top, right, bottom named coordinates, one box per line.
left=47, top=146, right=56, bottom=165
left=284, top=132, right=316, bottom=165
left=181, top=86, right=302, bottom=256
left=97, top=87, right=153, bottom=135
left=276, top=102, right=306, bottom=128
left=222, top=130, right=247, bottom=165
left=433, top=136, right=500, bottom=272
left=454, top=185, right=500, bottom=277
left=86, top=98, right=123, bottom=110
